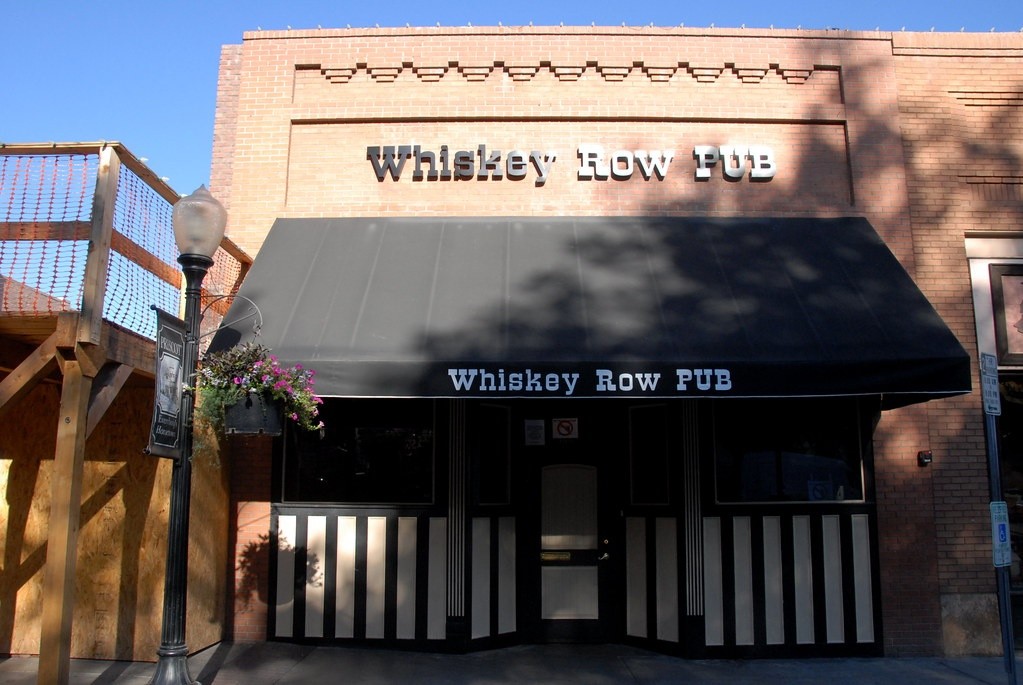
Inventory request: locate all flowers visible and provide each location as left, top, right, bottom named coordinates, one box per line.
left=181, top=342, right=323, bottom=430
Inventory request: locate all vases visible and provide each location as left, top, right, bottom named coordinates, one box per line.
left=225, top=387, right=285, bottom=436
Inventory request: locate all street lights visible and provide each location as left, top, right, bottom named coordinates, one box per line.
left=146, top=182, right=227, bottom=685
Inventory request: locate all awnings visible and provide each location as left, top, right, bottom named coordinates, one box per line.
left=200, top=215, right=971, bottom=426
